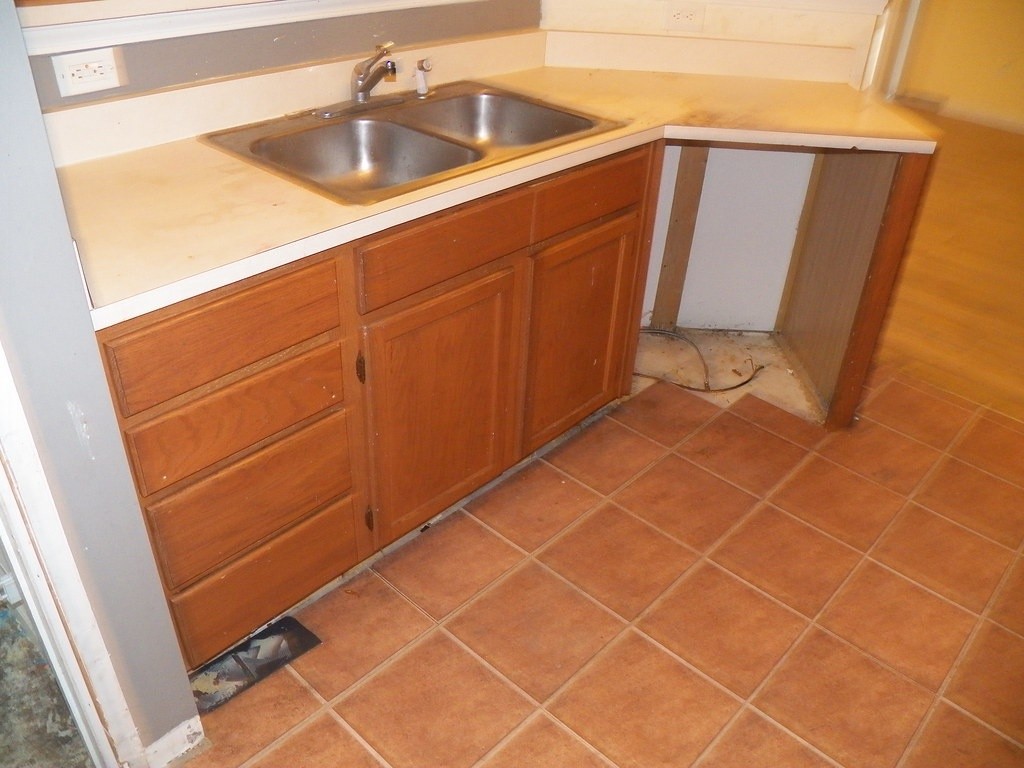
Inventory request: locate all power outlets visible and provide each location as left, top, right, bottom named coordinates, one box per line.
left=51, top=46, right=122, bottom=98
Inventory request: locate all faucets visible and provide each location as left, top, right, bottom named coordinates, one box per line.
left=349, top=40, right=398, bottom=105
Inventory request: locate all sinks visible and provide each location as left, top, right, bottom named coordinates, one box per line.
left=369, top=78, right=629, bottom=161
left=196, top=115, right=497, bottom=208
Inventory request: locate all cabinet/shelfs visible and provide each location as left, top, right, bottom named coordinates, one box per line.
left=355, top=125, right=665, bottom=560
left=87, top=243, right=368, bottom=678
